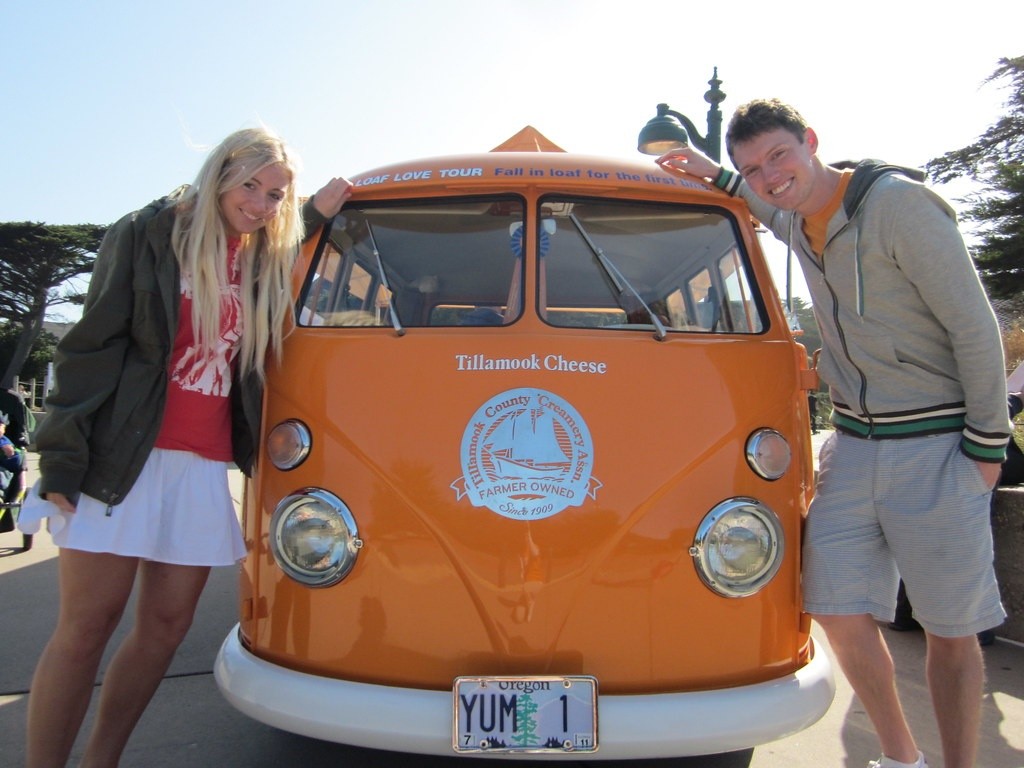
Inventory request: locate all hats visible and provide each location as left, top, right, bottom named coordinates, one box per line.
left=0, top=411, right=9, bottom=424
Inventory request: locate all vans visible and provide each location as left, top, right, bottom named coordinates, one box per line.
left=211, top=125, right=836, bottom=768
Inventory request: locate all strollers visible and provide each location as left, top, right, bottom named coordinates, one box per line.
left=0, top=386, right=32, bottom=549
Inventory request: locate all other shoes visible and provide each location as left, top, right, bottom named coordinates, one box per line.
left=867, top=757, right=929, bottom=768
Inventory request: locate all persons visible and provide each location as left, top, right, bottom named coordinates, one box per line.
left=0, top=410, right=27, bottom=471
left=16, top=127, right=354, bottom=768
left=6, top=383, right=36, bottom=450
left=654, top=103, right=1024, bottom=768
